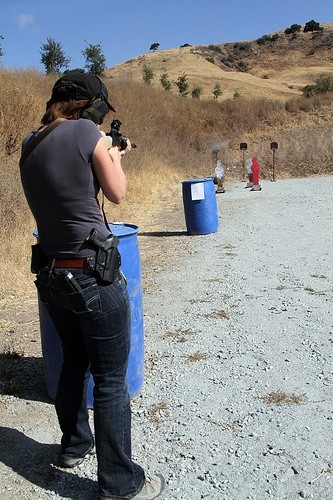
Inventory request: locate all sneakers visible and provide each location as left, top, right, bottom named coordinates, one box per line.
left=59, top=432, right=96, bottom=468
left=127, top=474, right=165, bottom=500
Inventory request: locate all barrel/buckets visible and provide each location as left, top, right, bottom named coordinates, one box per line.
left=181, top=178, right=218, bottom=235
left=33, top=222, right=145, bottom=409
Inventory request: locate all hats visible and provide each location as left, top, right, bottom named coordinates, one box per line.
left=51, top=72, right=116, bottom=112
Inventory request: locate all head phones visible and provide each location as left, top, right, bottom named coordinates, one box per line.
left=81, top=76, right=109, bottom=125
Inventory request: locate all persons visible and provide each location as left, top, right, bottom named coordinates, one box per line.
left=19, top=72, right=165, bottom=500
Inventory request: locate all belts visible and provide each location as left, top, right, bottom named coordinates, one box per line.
left=44, top=258, right=87, bottom=268
